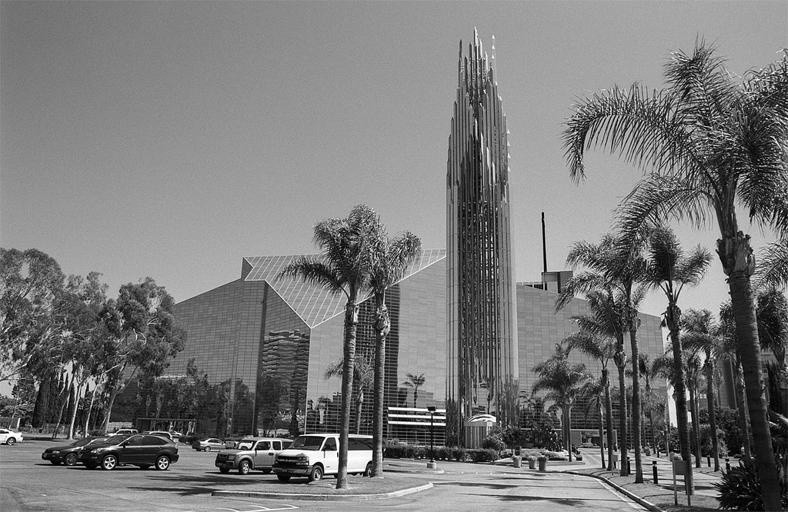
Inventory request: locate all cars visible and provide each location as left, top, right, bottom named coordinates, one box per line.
left=192, top=438, right=225, bottom=452
left=82, top=434, right=180, bottom=471
left=105, top=428, right=138, bottom=437
left=41, top=435, right=108, bottom=466
left=148, top=430, right=179, bottom=442
left=0, top=427, right=23, bottom=446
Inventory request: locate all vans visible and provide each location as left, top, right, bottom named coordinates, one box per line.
left=215, top=436, right=294, bottom=475
left=271, top=432, right=384, bottom=482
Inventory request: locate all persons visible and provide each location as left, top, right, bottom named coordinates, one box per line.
left=59, top=424, right=65, bottom=438
left=741, top=443, right=746, bottom=457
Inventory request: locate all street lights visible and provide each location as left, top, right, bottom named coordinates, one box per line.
left=427, top=406, right=437, bottom=463
